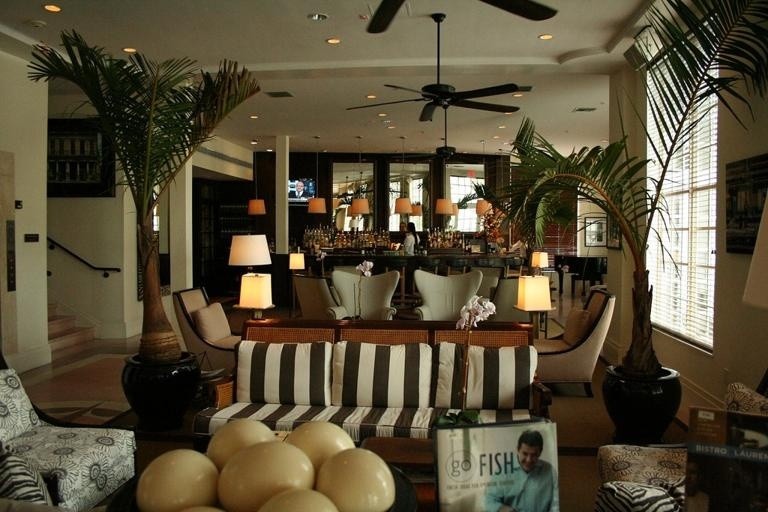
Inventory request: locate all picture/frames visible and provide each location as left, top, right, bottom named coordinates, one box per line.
left=605, top=184, right=622, bottom=251
left=584, top=216, right=607, bottom=248
left=46, top=117, right=117, bottom=199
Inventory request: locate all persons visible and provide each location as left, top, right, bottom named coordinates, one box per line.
left=403, top=222, right=420, bottom=254
left=685, top=462, right=709, bottom=512
left=288, top=180, right=313, bottom=199
left=481, top=429, right=557, bottom=512
left=511, top=231, right=534, bottom=267
left=398, top=222, right=406, bottom=234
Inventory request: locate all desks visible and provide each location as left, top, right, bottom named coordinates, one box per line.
left=351, top=433, right=437, bottom=469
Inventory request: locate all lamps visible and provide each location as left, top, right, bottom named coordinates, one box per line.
left=350, top=135, right=370, bottom=215
left=410, top=179, right=423, bottom=217
left=226, top=232, right=273, bottom=273
left=434, top=138, right=455, bottom=215
left=474, top=140, right=494, bottom=216
left=247, top=139, right=268, bottom=216
left=307, top=136, right=329, bottom=214
left=394, top=136, right=414, bottom=214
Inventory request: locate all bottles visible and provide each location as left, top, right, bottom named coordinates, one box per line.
left=423, top=226, right=471, bottom=253
left=303, top=223, right=391, bottom=249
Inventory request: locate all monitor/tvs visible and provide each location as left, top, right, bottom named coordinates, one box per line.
left=288, top=177, right=317, bottom=206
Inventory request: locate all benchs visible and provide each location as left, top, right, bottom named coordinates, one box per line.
left=187, top=317, right=555, bottom=485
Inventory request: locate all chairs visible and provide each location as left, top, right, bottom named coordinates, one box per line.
left=0, top=350, right=139, bottom=512
left=593, top=370, right=767, bottom=511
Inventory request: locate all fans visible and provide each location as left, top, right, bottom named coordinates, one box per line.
left=362, top=0, right=559, bottom=35
left=344, top=12, right=521, bottom=123
left=400, top=105, right=475, bottom=165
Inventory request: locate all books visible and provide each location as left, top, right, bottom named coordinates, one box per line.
left=432, top=416, right=559, bottom=512
left=685, top=406, right=767, bottom=512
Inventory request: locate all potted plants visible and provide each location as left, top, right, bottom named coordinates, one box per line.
left=23, top=25, right=268, bottom=433
left=456, top=2, right=768, bottom=442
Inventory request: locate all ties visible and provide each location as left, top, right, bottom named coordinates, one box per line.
left=297, top=191, right=300, bottom=198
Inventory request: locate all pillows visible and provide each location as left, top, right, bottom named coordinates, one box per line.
left=0, top=440, right=53, bottom=506
left=595, top=473, right=685, bottom=511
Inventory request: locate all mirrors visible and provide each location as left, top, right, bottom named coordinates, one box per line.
left=388, top=162, right=432, bottom=238
left=330, top=162, right=377, bottom=233
left=443, top=162, right=487, bottom=238
